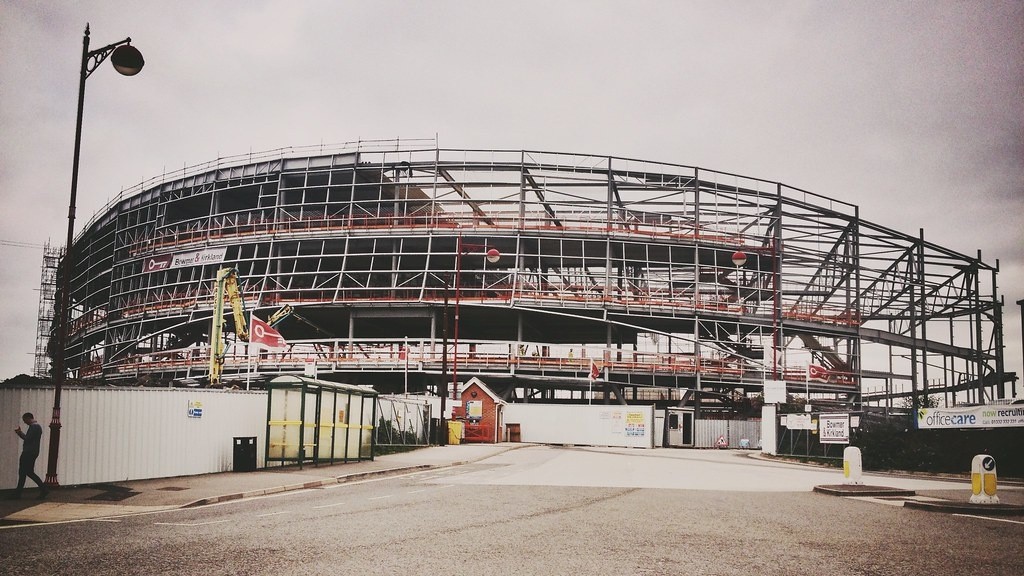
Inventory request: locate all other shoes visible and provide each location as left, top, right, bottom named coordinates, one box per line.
left=5, top=492, right=21, bottom=499
left=37, top=488, right=48, bottom=498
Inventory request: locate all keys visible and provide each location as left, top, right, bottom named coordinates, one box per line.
left=149, top=259, right=168, bottom=270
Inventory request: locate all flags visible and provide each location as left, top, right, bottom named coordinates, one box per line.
left=808, top=364, right=829, bottom=383
left=764, top=343, right=782, bottom=368
left=588, top=363, right=600, bottom=381
left=250, top=316, right=289, bottom=352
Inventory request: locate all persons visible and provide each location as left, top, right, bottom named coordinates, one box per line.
left=7, top=413, right=49, bottom=498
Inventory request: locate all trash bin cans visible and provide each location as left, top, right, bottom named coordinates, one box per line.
left=232, top=437, right=257, bottom=472
left=448, top=419, right=462, bottom=445
left=506, top=422, right=521, bottom=442
